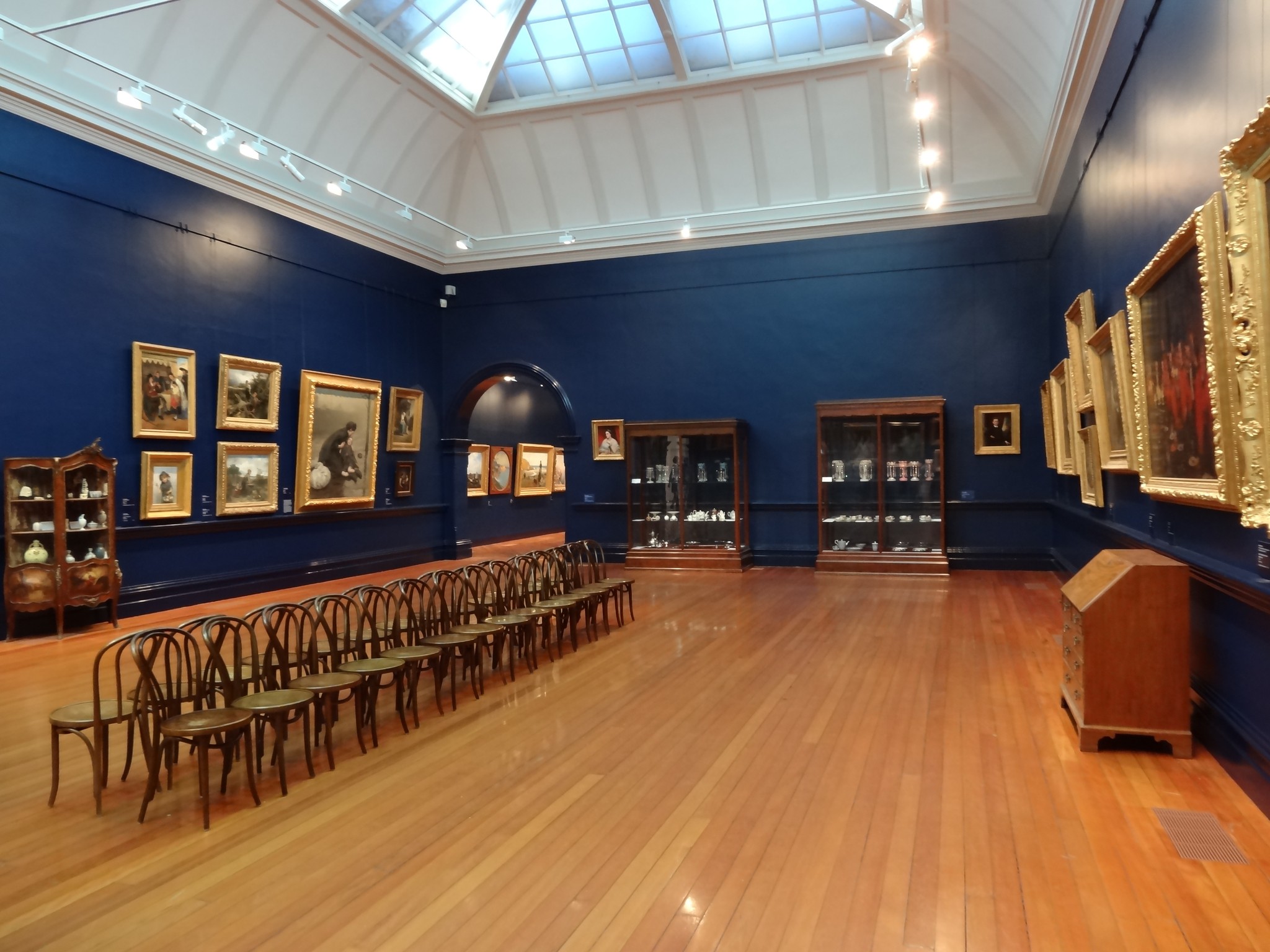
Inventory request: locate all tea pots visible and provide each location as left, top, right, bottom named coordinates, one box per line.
left=835, top=539, right=850, bottom=550
left=648, top=530, right=659, bottom=545
left=686, top=508, right=735, bottom=521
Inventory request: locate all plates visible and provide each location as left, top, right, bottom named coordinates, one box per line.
left=834, top=518, right=873, bottom=522
left=832, top=548, right=840, bottom=550
left=899, top=519, right=932, bottom=522
left=885, top=520, right=893, bottom=522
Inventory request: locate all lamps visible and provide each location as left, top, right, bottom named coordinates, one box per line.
left=682, top=218, right=692, bottom=237
left=557, top=230, right=576, bottom=244
left=395, top=206, right=412, bottom=220
left=117, top=82, right=353, bottom=196
left=456, top=235, right=473, bottom=249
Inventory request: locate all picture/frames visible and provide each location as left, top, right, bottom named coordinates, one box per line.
left=592, top=419, right=625, bottom=461
left=973, top=405, right=1020, bottom=453
left=1038, top=96, right=1270, bottom=531
left=386, top=385, right=424, bottom=453
left=294, top=370, right=383, bottom=514
left=215, top=354, right=282, bottom=432
left=140, top=450, right=194, bottom=521
left=132, top=341, right=196, bottom=441
left=394, top=460, right=417, bottom=498
left=216, top=441, right=279, bottom=516
left=467, top=443, right=566, bottom=496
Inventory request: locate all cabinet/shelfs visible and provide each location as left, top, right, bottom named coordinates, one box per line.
left=1061, top=549, right=1196, bottom=760
left=816, top=396, right=952, bottom=577
left=624, top=419, right=754, bottom=572
left=2, top=437, right=122, bottom=641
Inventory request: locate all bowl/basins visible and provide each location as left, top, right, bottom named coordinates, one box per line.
left=856, top=544, right=866, bottom=547
left=845, top=547, right=863, bottom=551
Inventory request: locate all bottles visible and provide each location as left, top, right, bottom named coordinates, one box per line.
left=66, top=550, right=75, bottom=563
left=23, top=540, right=48, bottom=563
left=85, top=543, right=108, bottom=560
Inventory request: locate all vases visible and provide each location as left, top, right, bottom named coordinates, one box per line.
left=96, top=542, right=105, bottom=560
left=24, top=540, right=49, bottom=563
left=66, top=548, right=75, bottom=564
left=84, top=547, right=96, bottom=561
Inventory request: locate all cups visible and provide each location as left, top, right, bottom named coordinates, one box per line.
left=832, top=545, right=838, bottom=549
left=899, top=515, right=930, bottom=520
left=885, top=517, right=891, bottom=520
left=835, top=515, right=872, bottom=520
left=872, top=541, right=878, bottom=551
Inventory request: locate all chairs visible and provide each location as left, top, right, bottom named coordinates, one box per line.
left=49, top=538, right=634, bottom=829
